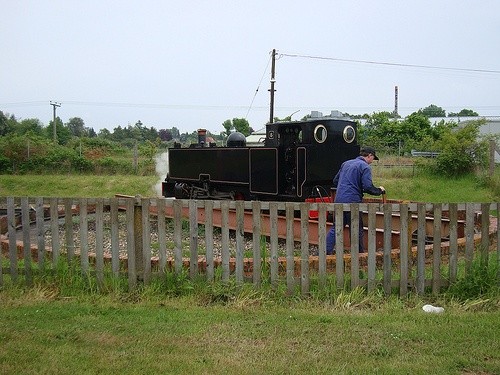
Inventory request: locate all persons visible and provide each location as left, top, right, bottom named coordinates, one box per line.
left=313, top=146, right=386, bottom=257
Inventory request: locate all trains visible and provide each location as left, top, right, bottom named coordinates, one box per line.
left=159, top=111, right=361, bottom=217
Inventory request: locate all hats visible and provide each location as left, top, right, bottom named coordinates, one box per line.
left=360, top=146, right=380, bottom=162
